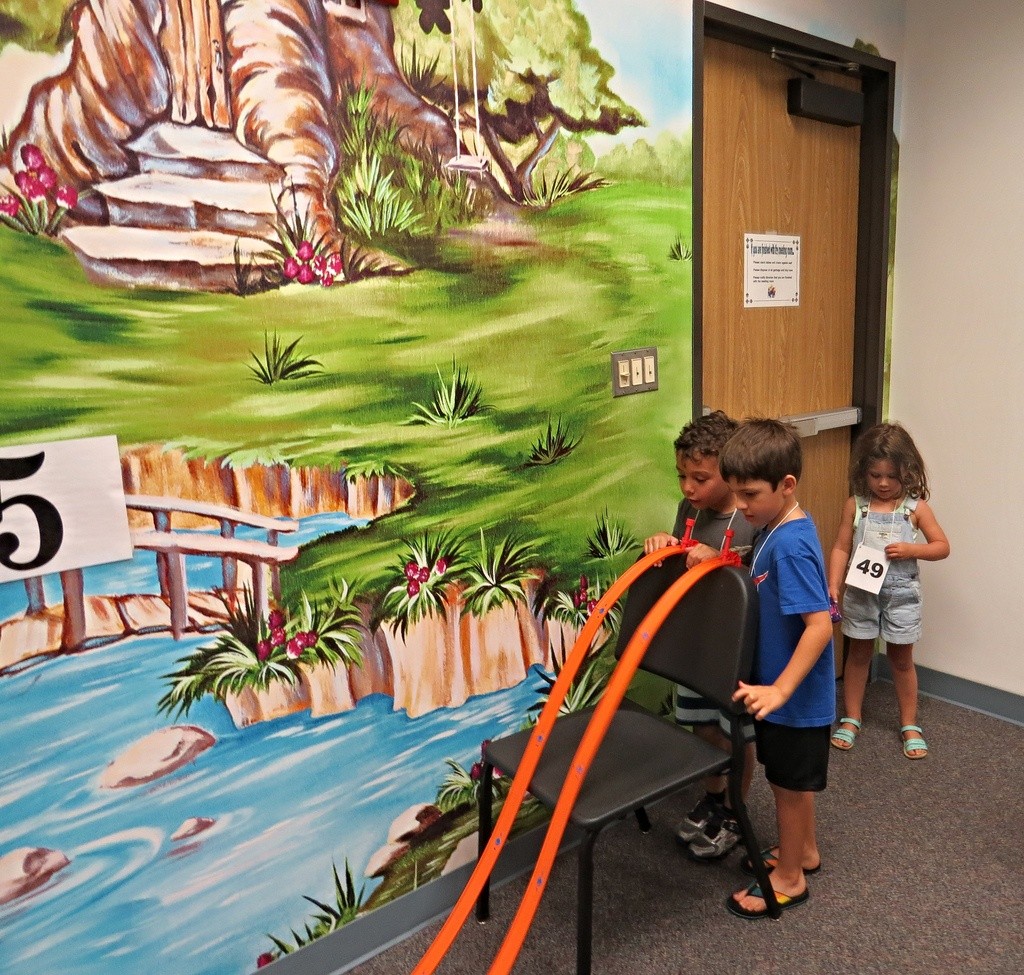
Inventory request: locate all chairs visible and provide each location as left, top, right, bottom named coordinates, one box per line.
left=479, top=553, right=781, bottom=974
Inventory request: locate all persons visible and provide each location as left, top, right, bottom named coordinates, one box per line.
left=718, top=417, right=836, bottom=918
left=827, top=423, right=950, bottom=758
left=645, top=410, right=764, bottom=860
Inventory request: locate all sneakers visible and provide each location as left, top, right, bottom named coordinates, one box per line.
left=687, top=810, right=749, bottom=863
left=675, top=795, right=726, bottom=846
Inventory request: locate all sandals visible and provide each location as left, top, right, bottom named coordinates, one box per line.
left=900, top=725, right=926, bottom=758
left=831, top=717, right=862, bottom=750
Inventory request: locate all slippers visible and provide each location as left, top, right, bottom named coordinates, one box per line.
left=740, top=845, right=821, bottom=874
left=726, top=875, right=808, bottom=920
